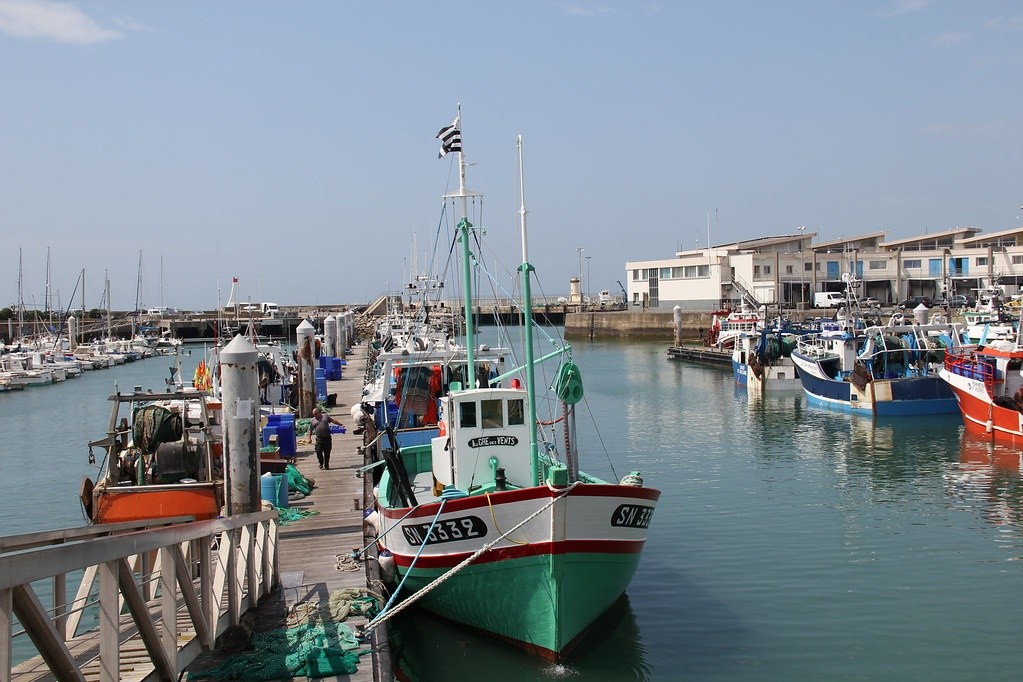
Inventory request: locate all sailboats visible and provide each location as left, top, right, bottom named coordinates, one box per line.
left=359, top=100, right=661, bottom=663
left=0, top=244, right=169, bottom=391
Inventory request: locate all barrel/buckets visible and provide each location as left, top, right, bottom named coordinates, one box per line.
left=260, top=473, right=289, bottom=508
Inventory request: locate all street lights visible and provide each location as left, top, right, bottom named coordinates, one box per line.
left=585, top=256, right=592, bottom=297
left=576, top=247, right=585, bottom=312
left=797, top=226, right=806, bottom=310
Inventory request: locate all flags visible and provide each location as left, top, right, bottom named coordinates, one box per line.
left=436, top=117, right=461, bottom=160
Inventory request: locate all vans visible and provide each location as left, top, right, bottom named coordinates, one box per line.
left=813, top=292, right=847, bottom=310
left=980, top=287, right=1005, bottom=305
left=1011, top=290, right=1023, bottom=301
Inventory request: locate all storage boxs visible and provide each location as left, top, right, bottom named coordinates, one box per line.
left=951, top=356, right=996, bottom=382
left=263, top=413, right=296, bottom=455
left=316, top=355, right=341, bottom=401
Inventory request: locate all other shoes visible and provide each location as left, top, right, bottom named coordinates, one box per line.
left=325, top=465, right=329, bottom=470
left=320, top=462, right=323, bottom=468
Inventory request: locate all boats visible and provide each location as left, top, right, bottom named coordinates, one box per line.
left=938, top=309, right=1023, bottom=451
left=705, top=267, right=965, bottom=416
left=152, top=274, right=298, bottom=466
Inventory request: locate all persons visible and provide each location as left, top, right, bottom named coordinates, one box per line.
left=308, top=408, right=346, bottom=470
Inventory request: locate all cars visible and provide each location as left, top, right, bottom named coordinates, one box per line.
left=896, top=296, right=934, bottom=309
left=939, top=294, right=976, bottom=309
left=854, top=297, right=881, bottom=309
